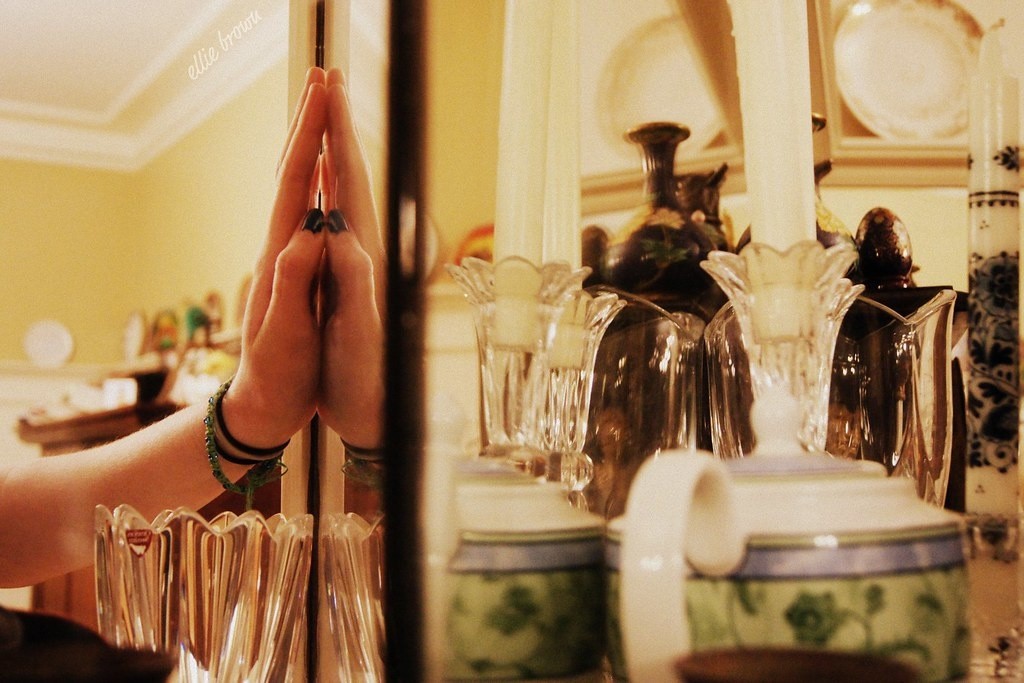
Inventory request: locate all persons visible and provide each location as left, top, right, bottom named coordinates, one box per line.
left=318, top=68, right=390, bottom=517
left=0, top=67, right=326, bottom=683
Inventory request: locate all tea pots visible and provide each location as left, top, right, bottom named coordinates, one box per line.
left=443, top=447, right=605, bottom=683
left=607, top=394, right=971, bottom=682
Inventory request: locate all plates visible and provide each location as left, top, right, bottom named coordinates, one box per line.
left=835, top=0, right=985, bottom=137
left=595, top=16, right=723, bottom=159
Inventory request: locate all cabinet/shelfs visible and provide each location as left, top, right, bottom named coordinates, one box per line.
left=20, top=398, right=281, bottom=640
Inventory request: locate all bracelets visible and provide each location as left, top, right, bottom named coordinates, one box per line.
left=341, top=438, right=384, bottom=492
left=205, top=378, right=291, bottom=495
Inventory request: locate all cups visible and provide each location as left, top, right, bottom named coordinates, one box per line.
left=320, top=512, right=382, bottom=683
left=444, top=283, right=704, bottom=520
left=93, top=503, right=313, bottom=683
left=699, top=282, right=957, bottom=509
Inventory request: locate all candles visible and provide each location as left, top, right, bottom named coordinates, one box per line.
left=725, top=0, right=817, bottom=347
left=486, top=1, right=586, bottom=369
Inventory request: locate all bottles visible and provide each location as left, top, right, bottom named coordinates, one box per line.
left=600, top=121, right=727, bottom=301
